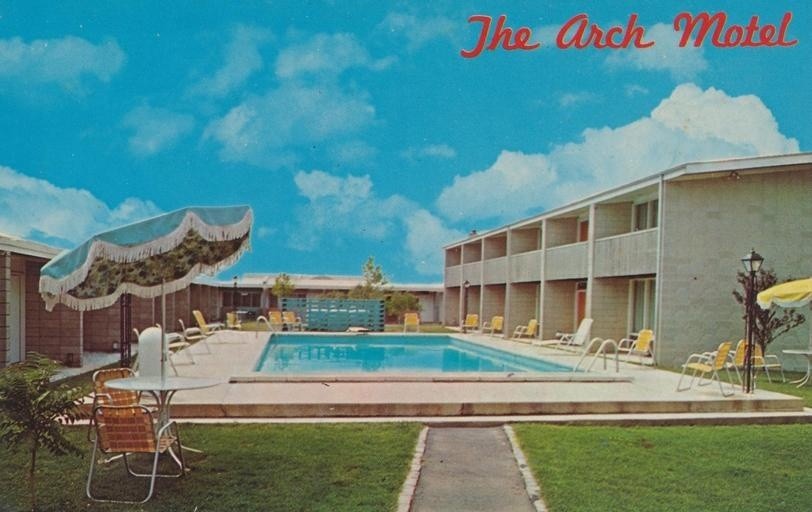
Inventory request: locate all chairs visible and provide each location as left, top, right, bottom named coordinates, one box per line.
left=403, top=312, right=421, bottom=334
left=675, top=339, right=788, bottom=398
left=511, top=317, right=538, bottom=341
left=554, top=316, right=594, bottom=354
left=460, top=312, right=479, bottom=334
left=613, top=330, right=657, bottom=367
left=481, top=315, right=505, bottom=339
left=84, top=310, right=303, bottom=506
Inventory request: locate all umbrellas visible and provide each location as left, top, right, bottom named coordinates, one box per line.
left=38, top=202, right=256, bottom=384
left=755, top=275, right=811, bottom=312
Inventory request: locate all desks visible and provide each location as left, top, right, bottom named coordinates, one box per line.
left=781, top=344, right=812, bottom=392
